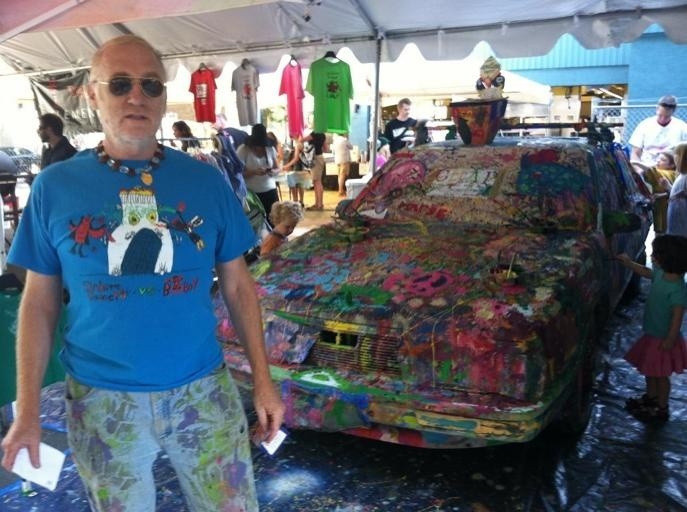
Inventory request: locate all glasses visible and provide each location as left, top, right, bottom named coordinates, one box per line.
left=91, top=76, right=164, bottom=98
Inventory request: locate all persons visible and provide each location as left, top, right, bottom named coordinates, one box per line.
left=630, top=150, right=676, bottom=232
left=1, top=35, right=285, bottom=512
left=627, top=96, right=687, bottom=177
left=374, top=99, right=422, bottom=155
left=36, top=113, right=78, bottom=170
left=259, top=201, right=303, bottom=257
left=172, top=98, right=352, bottom=215
left=657, top=144, right=687, bottom=236
left=615, top=234, right=687, bottom=422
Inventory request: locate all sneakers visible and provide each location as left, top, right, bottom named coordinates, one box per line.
left=306, top=191, right=347, bottom=211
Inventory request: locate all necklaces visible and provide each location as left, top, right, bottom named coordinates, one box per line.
left=94, top=140, right=164, bottom=177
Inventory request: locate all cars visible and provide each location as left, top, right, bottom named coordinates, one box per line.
left=0, top=145, right=38, bottom=176
left=208, top=95, right=657, bottom=460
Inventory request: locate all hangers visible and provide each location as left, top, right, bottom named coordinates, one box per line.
left=288, top=45, right=298, bottom=67
left=238, top=51, right=249, bottom=67
left=196, top=52, right=208, bottom=70
left=323, top=41, right=341, bottom=62
left=183, top=139, right=204, bottom=158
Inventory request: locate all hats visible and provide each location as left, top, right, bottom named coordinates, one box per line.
left=244, top=124, right=277, bottom=147
left=658, top=95, right=678, bottom=106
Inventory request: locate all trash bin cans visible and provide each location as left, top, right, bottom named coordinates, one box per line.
left=346, top=179, right=367, bottom=200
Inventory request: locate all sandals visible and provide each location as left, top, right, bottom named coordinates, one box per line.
left=625, top=393, right=670, bottom=422
